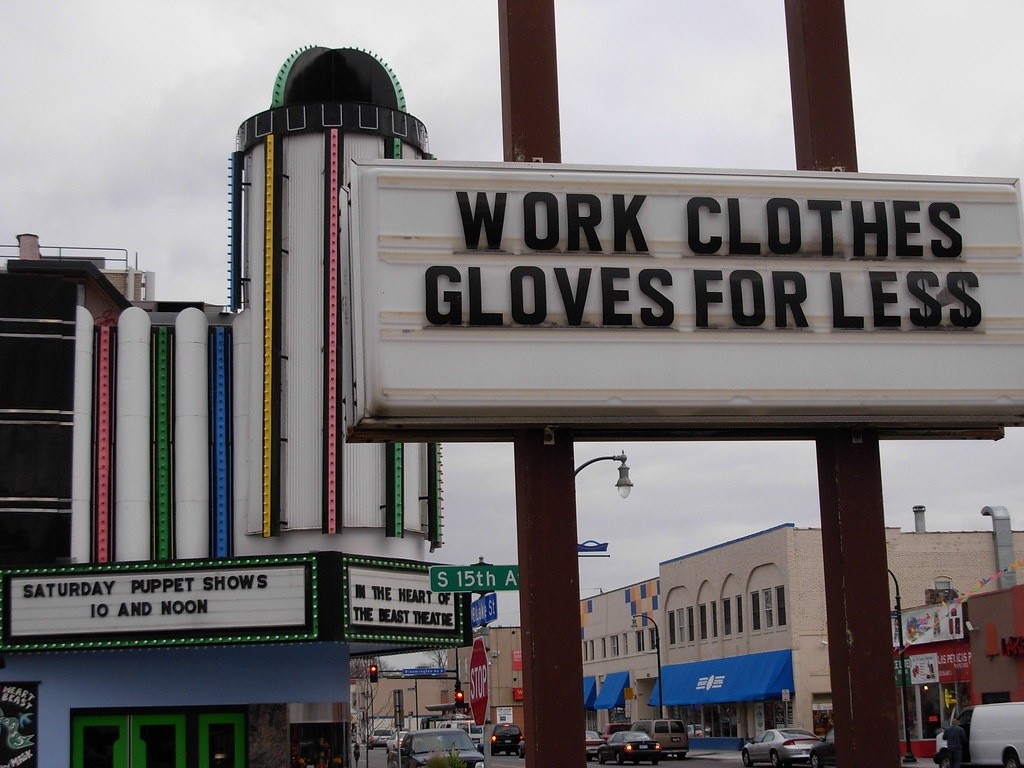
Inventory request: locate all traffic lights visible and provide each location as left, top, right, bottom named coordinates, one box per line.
left=455, top=690, right=464, bottom=707
left=369, top=665, right=378, bottom=682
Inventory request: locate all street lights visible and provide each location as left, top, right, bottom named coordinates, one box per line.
left=631, top=614, right=663, bottom=719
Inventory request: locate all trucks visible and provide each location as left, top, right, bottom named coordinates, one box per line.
left=436, top=721, right=484, bottom=749
left=933, top=702, right=1024, bottom=768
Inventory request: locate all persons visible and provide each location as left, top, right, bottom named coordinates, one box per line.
left=942, top=718, right=966, bottom=768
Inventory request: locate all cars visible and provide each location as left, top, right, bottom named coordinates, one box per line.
left=517, top=737, right=525, bottom=757
left=742, top=728, right=822, bottom=768
left=596, top=731, right=661, bottom=765
left=369, top=729, right=392, bottom=750
left=386, top=728, right=484, bottom=768
left=809, top=725, right=836, bottom=768
left=586, top=731, right=606, bottom=760
left=686, top=724, right=710, bottom=737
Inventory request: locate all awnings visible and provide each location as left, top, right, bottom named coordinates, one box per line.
left=646, top=649, right=796, bottom=706
left=583, top=676, right=597, bottom=708
left=593, top=671, right=630, bottom=710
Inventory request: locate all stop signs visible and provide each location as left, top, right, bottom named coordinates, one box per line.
left=469, top=635, right=488, bottom=728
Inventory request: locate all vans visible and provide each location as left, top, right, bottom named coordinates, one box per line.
left=483, top=723, right=522, bottom=756
left=630, top=720, right=689, bottom=760
left=599, top=723, right=633, bottom=742
